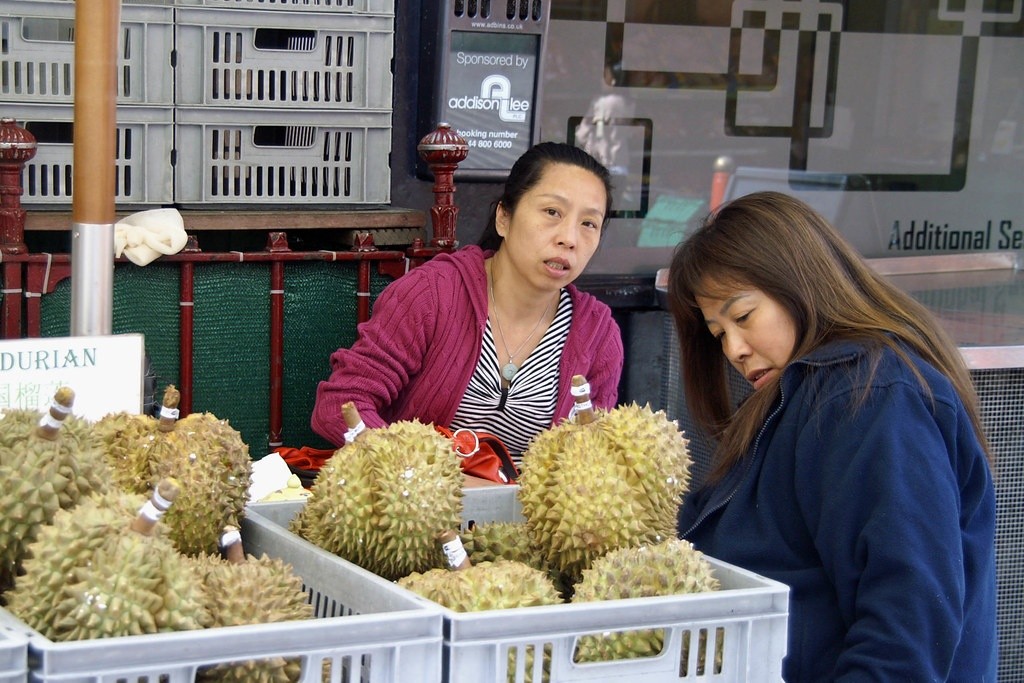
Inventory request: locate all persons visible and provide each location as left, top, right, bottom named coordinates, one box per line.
left=310, top=142, right=624, bottom=474
left=666, top=191, right=999, bottom=683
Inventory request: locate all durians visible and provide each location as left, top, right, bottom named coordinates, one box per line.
left=0, top=385, right=728, bottom=683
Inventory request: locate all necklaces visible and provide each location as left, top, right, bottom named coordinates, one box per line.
left=488, top=256, right=559, bottom=380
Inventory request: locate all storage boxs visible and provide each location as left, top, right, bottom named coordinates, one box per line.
left=242, top=484, right=791, bottom=683
left=0, top=507, right=446, bottom=682
left=1, top=1, right=394, bottom=204
